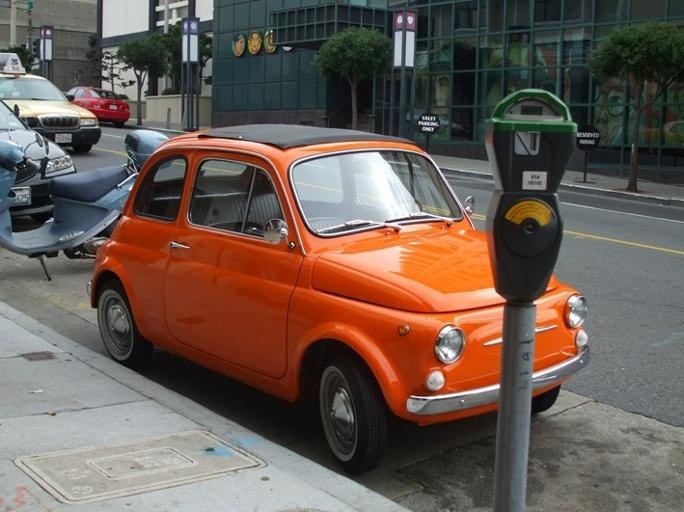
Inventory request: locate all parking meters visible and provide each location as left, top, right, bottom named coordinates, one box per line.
left=482, top=91, right=585, bottom=512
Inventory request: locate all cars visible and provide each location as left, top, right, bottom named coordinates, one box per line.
left=1, top=52, right=103, bottom=156
left=62, top=88, right=129, bottom=124
left=84, top=117, right=594, bottom=472
left=0, top=100, right=76, bottom=182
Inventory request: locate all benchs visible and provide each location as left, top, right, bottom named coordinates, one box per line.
left=153, top=194, right=282, bottom=226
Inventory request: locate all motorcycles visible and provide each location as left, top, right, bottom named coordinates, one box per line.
left=0, top=129, right=171, bottom=279
